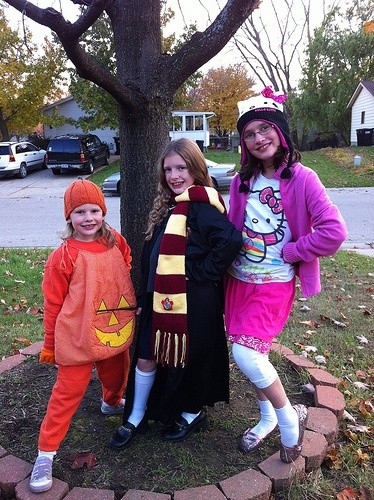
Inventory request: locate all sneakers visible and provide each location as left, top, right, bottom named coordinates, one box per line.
left=30, top=456, right=53, bottom=493
left=100, top=399, right=126, bottom=414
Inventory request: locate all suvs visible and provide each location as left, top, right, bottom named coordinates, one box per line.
left=45, top=134, right=110, bottom=175
left=0, top=142, right=48, bottom=179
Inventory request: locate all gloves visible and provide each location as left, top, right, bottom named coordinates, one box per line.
left=39, top=346, right=58, bottom=365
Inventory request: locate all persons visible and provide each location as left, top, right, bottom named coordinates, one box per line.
left=29, top=175, right=138, bottom=493
left=107, top=137, right=244, bottom=450
left=223, top=84, right=347, bottom=465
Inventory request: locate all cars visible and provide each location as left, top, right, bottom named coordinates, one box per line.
left=101, top=158, right=238, bottom=196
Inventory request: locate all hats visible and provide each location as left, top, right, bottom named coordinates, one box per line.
left=236, top=86, right=294, bottom=193
left=64, top=177, right=106, bottom=221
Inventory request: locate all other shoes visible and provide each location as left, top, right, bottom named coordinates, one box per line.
left=280, top=404, right=307, bottom=463
left=239, top=424, right=279, bottom=452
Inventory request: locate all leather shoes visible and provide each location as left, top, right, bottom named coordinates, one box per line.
left=161, top=408, right=208, bottom=442
left=109, top=415, right=148, bottom=451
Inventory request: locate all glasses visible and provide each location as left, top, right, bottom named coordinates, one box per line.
left=242, top=124, right=274, bottom=142
